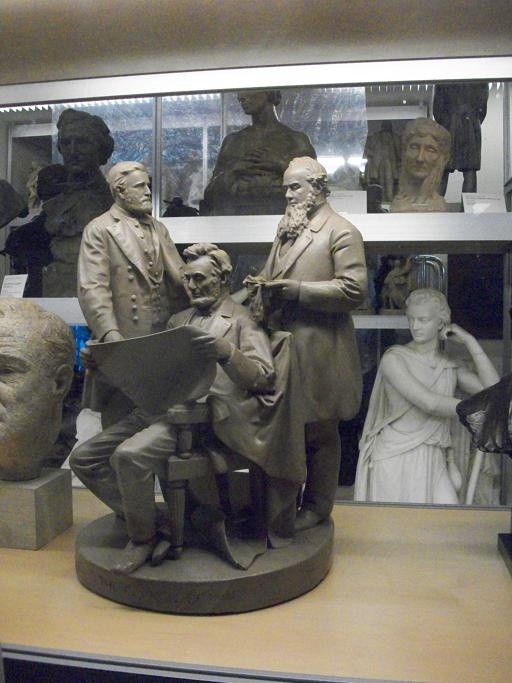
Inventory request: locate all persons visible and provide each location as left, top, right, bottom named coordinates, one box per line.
left=1, top=85, right=512, bottom=574
